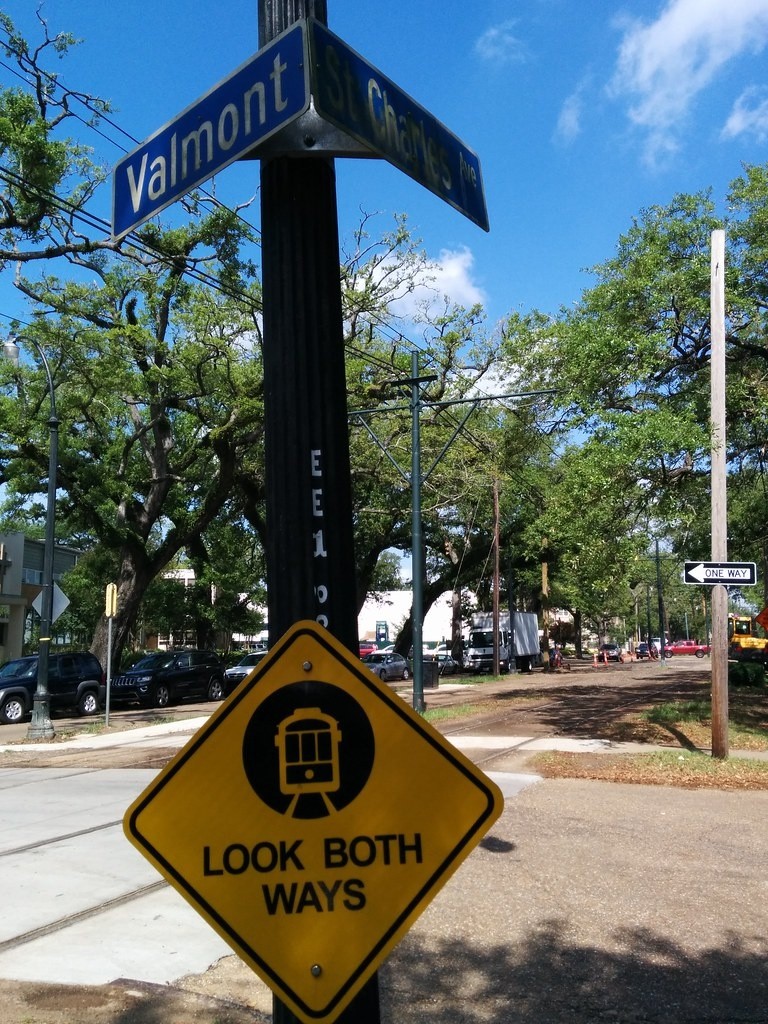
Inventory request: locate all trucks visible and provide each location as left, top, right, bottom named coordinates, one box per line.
left=466, top=611, right=540, bottom=676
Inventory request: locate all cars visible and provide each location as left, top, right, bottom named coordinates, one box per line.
left=359, top=643, right=378, bottom=658
left=371, top=644, right=394, bottom=654
left=635, top=643, right=659, bottom=660
left=423, top=643, right=452, bottom=657
left=424, top=654, right=459, bottom=675
left=224, top=651, right=270, bottom=693
left=362, top=653, right=410, bottom=683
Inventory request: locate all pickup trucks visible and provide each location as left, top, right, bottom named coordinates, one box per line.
left=659, top=640, right=712, bottom=658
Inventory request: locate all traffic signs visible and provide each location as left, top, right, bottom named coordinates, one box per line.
left=683, top=561, right=758, bottom=585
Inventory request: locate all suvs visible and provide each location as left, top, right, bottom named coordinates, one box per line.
left=111, top=650, right=226, bottom=708
left=598, top=644, right=622, bottom=662
left=0, top=650, right=106, bottom=724
